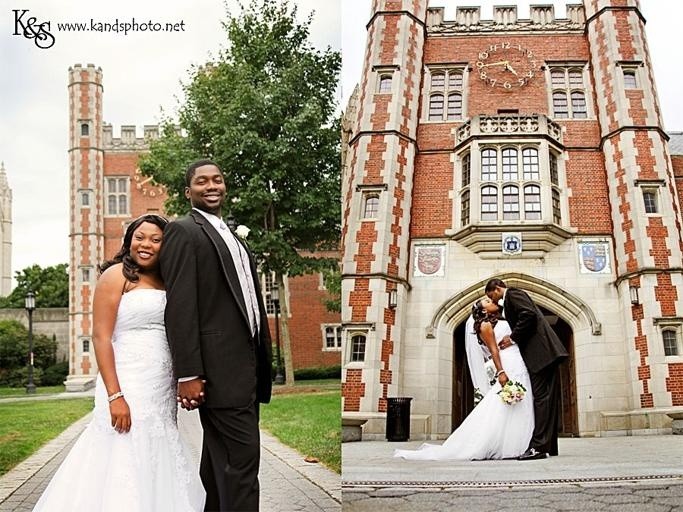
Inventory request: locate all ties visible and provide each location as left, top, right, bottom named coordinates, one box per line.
left=218, top=220, right=262, bottom=346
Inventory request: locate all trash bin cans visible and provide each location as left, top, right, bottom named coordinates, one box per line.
left=386, top=397, right=413, bottom=442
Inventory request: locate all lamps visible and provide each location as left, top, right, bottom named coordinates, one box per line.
left=390, top=289, right=397, bottom=309
left=629, top=285, right=639, bottom=305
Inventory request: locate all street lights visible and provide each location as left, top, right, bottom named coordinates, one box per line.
left=267, top=282, right=286, bottom=385
left=23, top=289, right=43, bottom=395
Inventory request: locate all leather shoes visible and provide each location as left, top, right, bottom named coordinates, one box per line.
left=516, top=448, right=546, bottom=460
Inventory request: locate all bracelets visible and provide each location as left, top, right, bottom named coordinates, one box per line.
left=508, top=337, right=516, bottom=345
left=107, top=391, right=122, bottom=405
left=496, top=369, right=505, bottom=375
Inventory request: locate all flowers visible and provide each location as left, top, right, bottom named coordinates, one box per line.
left=235, top=225, right=250, bottom=245
left=497, top=382, right=526, bottom=405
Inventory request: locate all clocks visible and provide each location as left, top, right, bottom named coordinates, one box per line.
left=476, top=42, right=538, bottom=90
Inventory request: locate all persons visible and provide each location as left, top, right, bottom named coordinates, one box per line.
left=390, top=296, right=534, bottom=461
left=27, top=214, right=210, bottom=512
left=156, top=160, right=272, bottom=512
left=485, top=278, right=569, bottom=460
